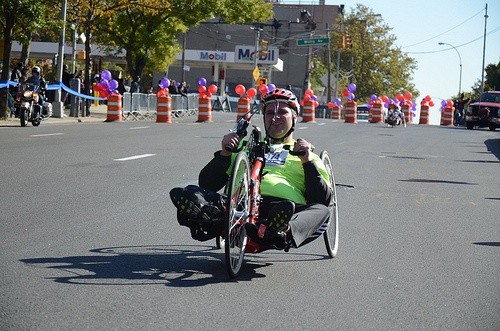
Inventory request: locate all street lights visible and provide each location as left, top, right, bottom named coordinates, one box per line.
left=439, top=42, right=462, bottom=98
left=326, top=13, right=382, bottom=102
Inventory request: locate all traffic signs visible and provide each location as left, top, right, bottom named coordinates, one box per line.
left=297, top=37, right=330, bottom=45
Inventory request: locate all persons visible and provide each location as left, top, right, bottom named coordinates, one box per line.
left=0, top=62, right=85, bottom=117
left=456, top=97, right=471, bottom=128
left=169, top=88, right=332, bottom=249
left=388, top=100, right=406, bottom=127
left=119, top=75, right=190, bottom=97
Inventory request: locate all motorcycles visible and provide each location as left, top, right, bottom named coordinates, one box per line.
left=16, top=79, right=53, bottom=127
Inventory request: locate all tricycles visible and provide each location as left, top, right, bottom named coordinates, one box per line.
left=387, top=109, right=406, bottom=129
left=217, top=97, right=339, bottom=279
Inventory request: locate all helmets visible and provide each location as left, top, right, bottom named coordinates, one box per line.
left=262, top=88, right=300, bottom=114
left=32, top=66, right=41, bottom=75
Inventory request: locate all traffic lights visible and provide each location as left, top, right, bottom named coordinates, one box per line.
left=261, top=40, right=268, bottom=57
left=345, top=34, right=352, bottom=49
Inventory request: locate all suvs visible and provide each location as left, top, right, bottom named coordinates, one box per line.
left=467, top=91, right=500, bottom=131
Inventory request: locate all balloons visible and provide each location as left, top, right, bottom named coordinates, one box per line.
left=300, top=82, right=434, bottom=117
left=441, top=100, right=447, bottom=106
left=197, top=77, right=276, bottom=98
left=94, top=69, right=180, bottom=97
left=447, top=100, right=454, bottom=106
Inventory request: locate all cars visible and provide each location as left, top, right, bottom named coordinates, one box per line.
left=357, top=106, right=370, bottom=122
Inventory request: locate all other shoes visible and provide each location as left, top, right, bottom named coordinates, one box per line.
left=259, top=201, right=295, bottom=238
left=170, top=187, right=219, bottom=227
